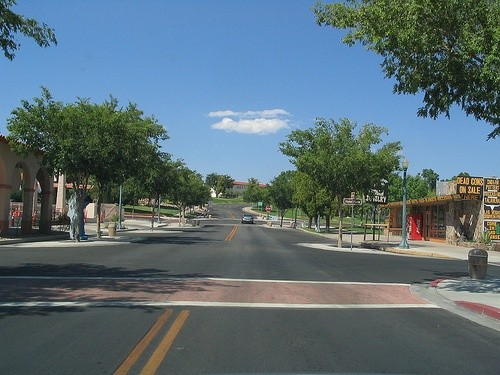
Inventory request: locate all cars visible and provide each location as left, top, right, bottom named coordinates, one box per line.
left=242, top=214, right=253, bottom=223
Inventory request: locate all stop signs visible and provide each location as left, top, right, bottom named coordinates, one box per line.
left=266, top=205, right=271, bottom=212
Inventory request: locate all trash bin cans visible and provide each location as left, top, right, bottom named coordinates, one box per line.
left=468, top=249, right=488, bottom=279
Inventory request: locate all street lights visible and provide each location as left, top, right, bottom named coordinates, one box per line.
left=399, top=159, right=409, bottom=249
